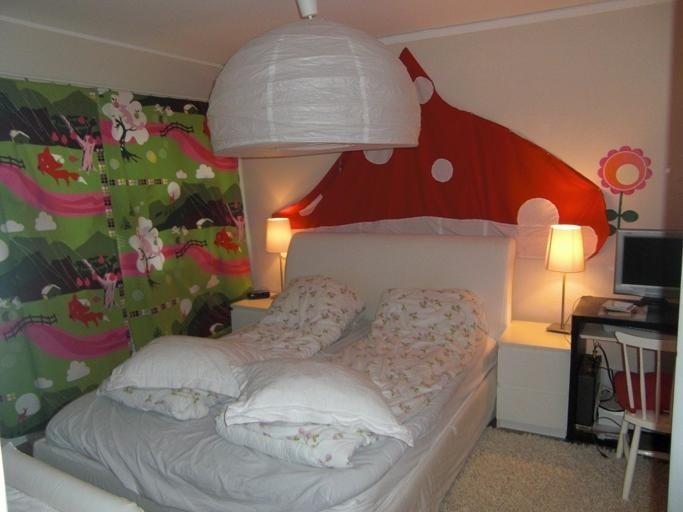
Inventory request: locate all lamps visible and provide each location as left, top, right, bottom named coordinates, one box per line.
left=264, top=217, right=293, bottom=298
left=544, top=224, right=585, bottom=335
left=203, top=1, right=423, bottom=158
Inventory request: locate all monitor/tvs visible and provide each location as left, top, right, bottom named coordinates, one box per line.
left=613, top=229, right=683, bottom=306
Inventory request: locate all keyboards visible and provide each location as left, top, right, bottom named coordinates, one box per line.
left=603, top=324, right=676, bottom=339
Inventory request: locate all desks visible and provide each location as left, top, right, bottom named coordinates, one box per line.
left=567, top=295, right=679, bottom=441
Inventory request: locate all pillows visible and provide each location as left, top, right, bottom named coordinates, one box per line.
left=211, top=412, right=377, bottom=470
left=103, top=387, right=221, bottom=421
left=105, top=332, right=242, bottom=399
left=223, top=358, right=415, bottom=448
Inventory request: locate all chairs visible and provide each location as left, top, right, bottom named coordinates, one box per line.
left=613, top=331, right=678, bottom=500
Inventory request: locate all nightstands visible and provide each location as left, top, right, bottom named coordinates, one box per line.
left=494, top=319, right=571, bottom=439
left=229, top=296, right=274, bottom=334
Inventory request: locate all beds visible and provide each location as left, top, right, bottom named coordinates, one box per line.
left=32, top=232, right=515, bottom=512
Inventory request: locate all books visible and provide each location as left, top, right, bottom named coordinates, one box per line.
left=601, top=299, right=633, bottom=311
left=598, top=305, right=647, bottom=322
left=607, top=305, right=639, bottom=317
